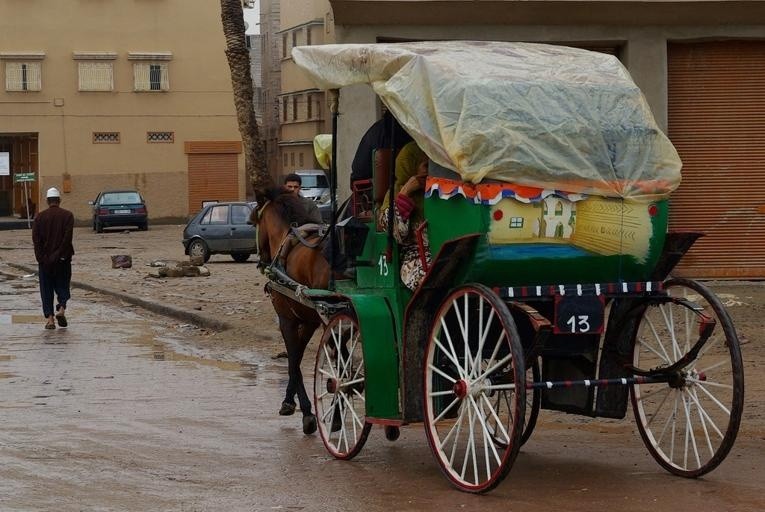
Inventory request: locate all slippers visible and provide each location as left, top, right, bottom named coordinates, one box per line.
left=45, top=321, right=55, bottom=329
left=56, top=314, right=67, bottom=329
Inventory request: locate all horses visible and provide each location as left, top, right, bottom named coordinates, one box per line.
left=249, top=174, right=401, bottom=443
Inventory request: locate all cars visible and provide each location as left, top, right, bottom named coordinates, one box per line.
left=183, top=201, right=257, bottom=263
left=87, top=190, right=148, bottom=233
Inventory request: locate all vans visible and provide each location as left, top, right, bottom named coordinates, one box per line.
left=292, top=169, right=332, bottom=211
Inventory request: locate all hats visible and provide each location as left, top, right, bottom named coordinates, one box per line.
left=47, top=188, right=60, bottom=198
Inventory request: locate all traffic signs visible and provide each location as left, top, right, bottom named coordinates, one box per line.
left=15, top=173, right=36, bottom=181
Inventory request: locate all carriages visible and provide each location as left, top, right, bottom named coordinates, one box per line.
left=248, top=42, right=744, bottom=493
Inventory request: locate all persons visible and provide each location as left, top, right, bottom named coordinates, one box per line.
left=380, top=139, right=426, bottom=214
left=284, top=174, right=324, bottom=224
left=32, top=187, right=75, bottom=330
left=18, top=198, right=36, bottom=219
left=319, top=110, right=415, bottom=279
